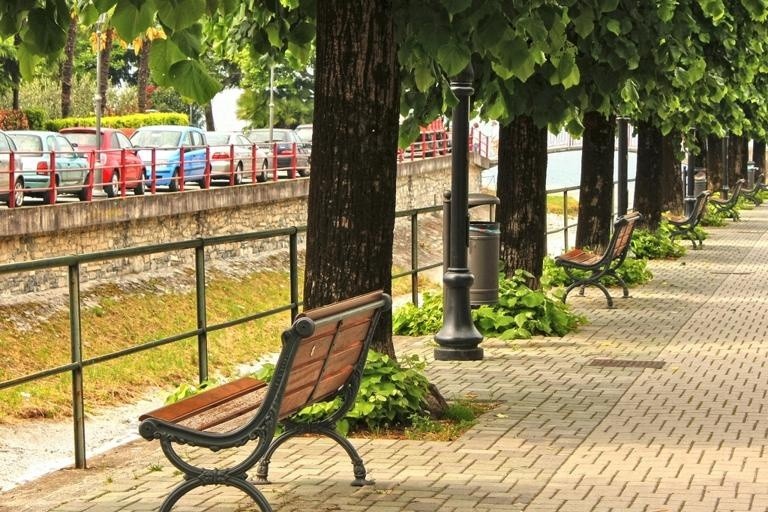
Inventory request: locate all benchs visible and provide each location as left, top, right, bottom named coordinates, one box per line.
left=666, top=173, right=765, bottom=250
left=137, top=288, right=392, bottom=512
left=553, top=211, right=642, bottom=309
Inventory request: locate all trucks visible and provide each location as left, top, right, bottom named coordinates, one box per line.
left=405, top=116, right=445, bottom=156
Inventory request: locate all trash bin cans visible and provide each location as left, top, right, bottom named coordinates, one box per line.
left=747, top=161, right=755, bottom=189
left=694, top=167, right=706, bottom=196
left=467, top=193, right=501, bottom=306
